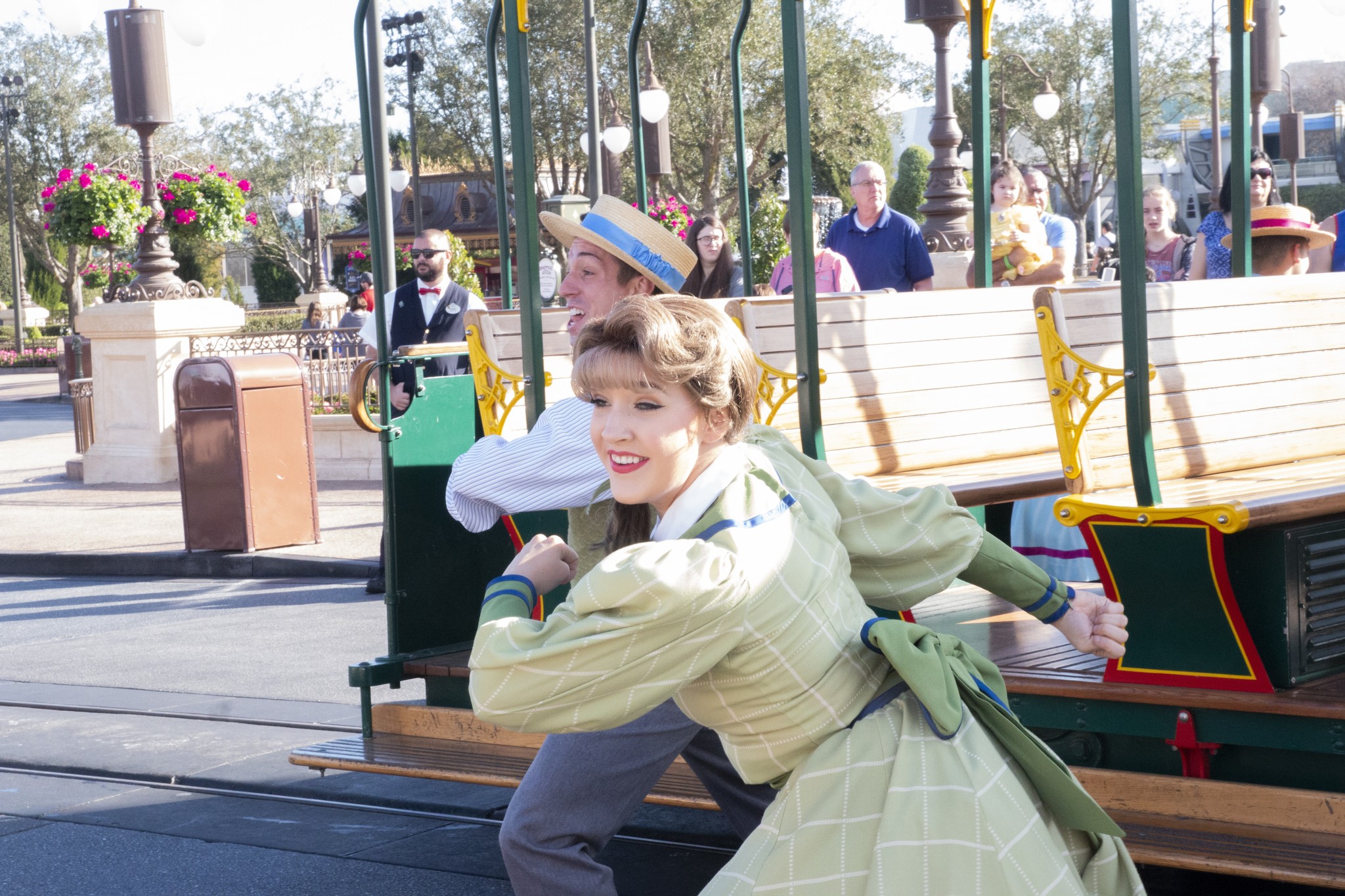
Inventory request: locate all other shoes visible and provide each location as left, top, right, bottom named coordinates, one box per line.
left=366, top=567, right=386, bottom=592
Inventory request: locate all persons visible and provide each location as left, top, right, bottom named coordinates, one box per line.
left=1306, top=128, right=1345, bottom=274
left=825, top=161, right=934, bottom=292
left=1220, top=204, right=1336, bottom=277
left=337, top=294, right=372, bottom=359
left=768, top=207, right=861, bottom=294
left=1188, top=147, right=1284, bottom=281
left=752, top=283, right=776, bottom=296
left=1091, top=222, right=1117, bottom=272
left=1086, top=243, right=1094, bottom=259
left=356, top=271, right=375, bottom=312
left=355, top=229, right=488, bottom=595
left=301, top=302, right=331, bottom=360
left=677, top=215, right=745, bottom=299
left=466, top=295, right=1146, bottom=896
left=965, top=159, right=1077, bottom=288
left=1142, top=185, right=1196, bottom=282
left=447, top=194, right=776, bottom=896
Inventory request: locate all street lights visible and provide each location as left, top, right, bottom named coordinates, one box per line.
left=0, top=75, right=28, bottom=357
left=288, top=161, right=342, bottom=287
left=579, top=77, right=631, bottom=209
left=347, top=138, right=410, bottom=238
left=638, top=38, right=674, bottom=228
left=1207, top=0, right=1287, bottom=213
left=1000, top=53, right=1061, bottom=159
left=380, top=11, right=428, bottom=239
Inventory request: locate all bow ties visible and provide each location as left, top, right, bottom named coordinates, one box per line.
left=418, top=288, right=441, bottom=295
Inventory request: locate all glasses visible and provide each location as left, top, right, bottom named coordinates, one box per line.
left=410, top=248, right=446, bottom=259
left=695, top=235, right=726, bottom=246
left=1027, top=187, right=1048, bottom=197
left=852, top=179, right=887, bottom=189
left=1250, top=168, right=1274, bottom=181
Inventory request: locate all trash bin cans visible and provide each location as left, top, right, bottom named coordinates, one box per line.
left=55, top=335, right=91, bottom=395
left=173, top=352, right=322, bottom=553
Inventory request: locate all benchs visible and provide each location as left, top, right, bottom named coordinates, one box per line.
left=1034, top=271, right=1344, bottom=529
left=463, top=297, right=726, bottom=442
left=726, top=287, right=1065, bottom=505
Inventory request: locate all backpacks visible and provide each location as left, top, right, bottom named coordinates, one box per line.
left=1102, top=235, right=1119, bottom=259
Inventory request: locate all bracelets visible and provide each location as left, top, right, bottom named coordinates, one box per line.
left=1004, top=255, right=1014, bottom=269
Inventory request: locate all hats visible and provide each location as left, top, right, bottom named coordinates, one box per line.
left=356, top=272, right=373, bottom=283
left=1220, top=206, right=1337, bottom=251
left=540, top=194, right=698, bottom=295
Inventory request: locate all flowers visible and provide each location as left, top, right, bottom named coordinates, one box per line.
left=37, top=162, right=258, bottom=288
left=347, top=242, right=412, bottom=271
left=631, top=194, right=694, bottom=242
